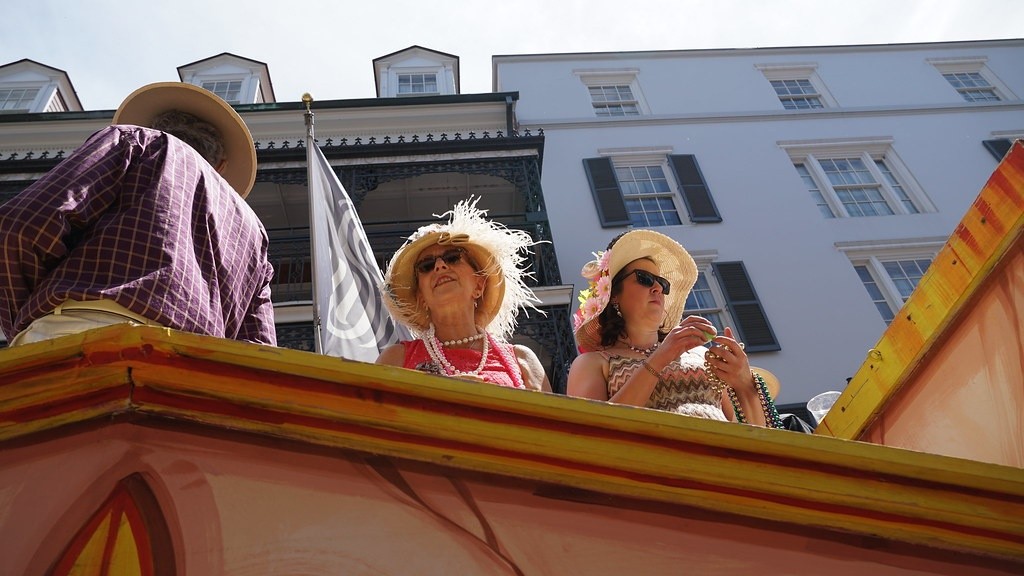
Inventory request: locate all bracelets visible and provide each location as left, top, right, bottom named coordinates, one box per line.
left=641, top=362, right=662, bottom=379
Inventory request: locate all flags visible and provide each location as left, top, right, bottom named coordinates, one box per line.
left=309, top=143, right=416, bottom=366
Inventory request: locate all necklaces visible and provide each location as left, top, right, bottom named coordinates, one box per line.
left=421, top=324, right=524, bottom=390
left=703, top=342, right=784, bottom=432
left=625, top=342, right=660, bottom=356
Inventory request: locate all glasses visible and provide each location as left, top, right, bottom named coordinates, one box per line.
left=414, top=247, right=480, bottom=288
left=611, top=269, right=671, bottom=295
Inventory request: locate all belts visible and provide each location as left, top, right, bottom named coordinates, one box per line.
left=39, top=298, right=164, bottom=327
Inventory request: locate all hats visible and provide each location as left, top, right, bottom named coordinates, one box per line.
left=110, top=81, right=257, bottom=200
left=572, top=229, right=699, bottom=352
left=749, top=366, right=780, bottom=402
left=377, top=194, right=552, bottom=344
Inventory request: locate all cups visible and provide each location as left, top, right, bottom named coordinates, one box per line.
left=807, top=391, right=842, bottom=424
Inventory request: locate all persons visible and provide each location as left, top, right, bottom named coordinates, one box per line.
left=374, top=230, right=553, bottom=395
left=566, top=230, right=768, bottom=433
left=0, top=82, right=277, bottom=353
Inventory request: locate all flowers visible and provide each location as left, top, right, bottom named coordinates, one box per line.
left=573, top=249, right=612, bottom=354
